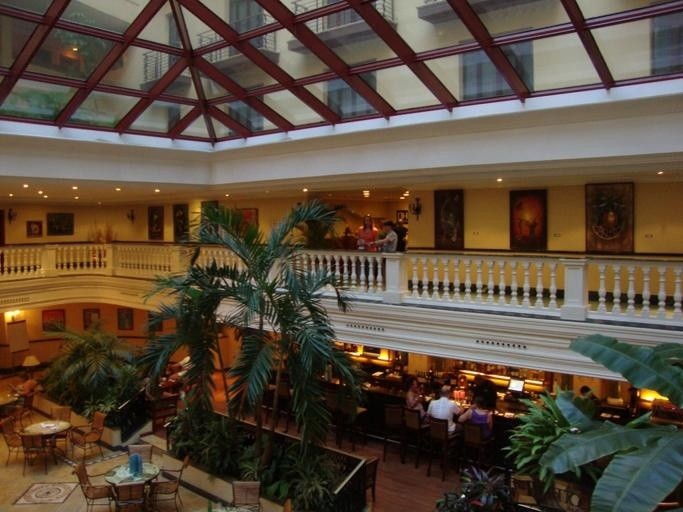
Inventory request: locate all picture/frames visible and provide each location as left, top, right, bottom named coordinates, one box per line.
left=41, top=309, right=66, bottom=333
left=83, top=308, right=102, bottom=332
left=172, top=204, right=190, bottom=243
left=233, top=207, right=259, bottom=238
left=148, top=205, right=165, bottom=241
left=47, top=212, right=75, bottom=236
left=26, top=220, right=43, bottom=238
left=147, top=311, right=164, bottom=332
left=201, top=199, right=219, bottom=237
left=116, top=307, right=135, bottom=330
left=396, top=210, right=408, bottom=225
left=509, top=189, right=549, bottom=251
left=585, top=182, right=636, bottom=256
left=434, top=187, right=465, bottom=251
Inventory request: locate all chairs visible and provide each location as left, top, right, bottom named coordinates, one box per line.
left=0, top=404, right=192, bottom=512
left=283, top=498, right=292, bottom=512
left=263, top=383, right=520, bottom=483
left=231, top=481, right=261, bottom=512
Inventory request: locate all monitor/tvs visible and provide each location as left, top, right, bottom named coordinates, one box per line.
left=507, top=377, right=524, bottom=393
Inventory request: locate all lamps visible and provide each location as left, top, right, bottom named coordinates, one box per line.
left=21, top=355, right=40, bottom=379
left=409, top=198, right=422, bottom=220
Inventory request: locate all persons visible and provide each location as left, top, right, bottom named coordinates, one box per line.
left=580, top=385, right=597, bottom=400
left=359, top=214, right=398, bottom=283
left=168, top=348, right=196, bottom=380
left=405, top=374, right=497, bottom=442
